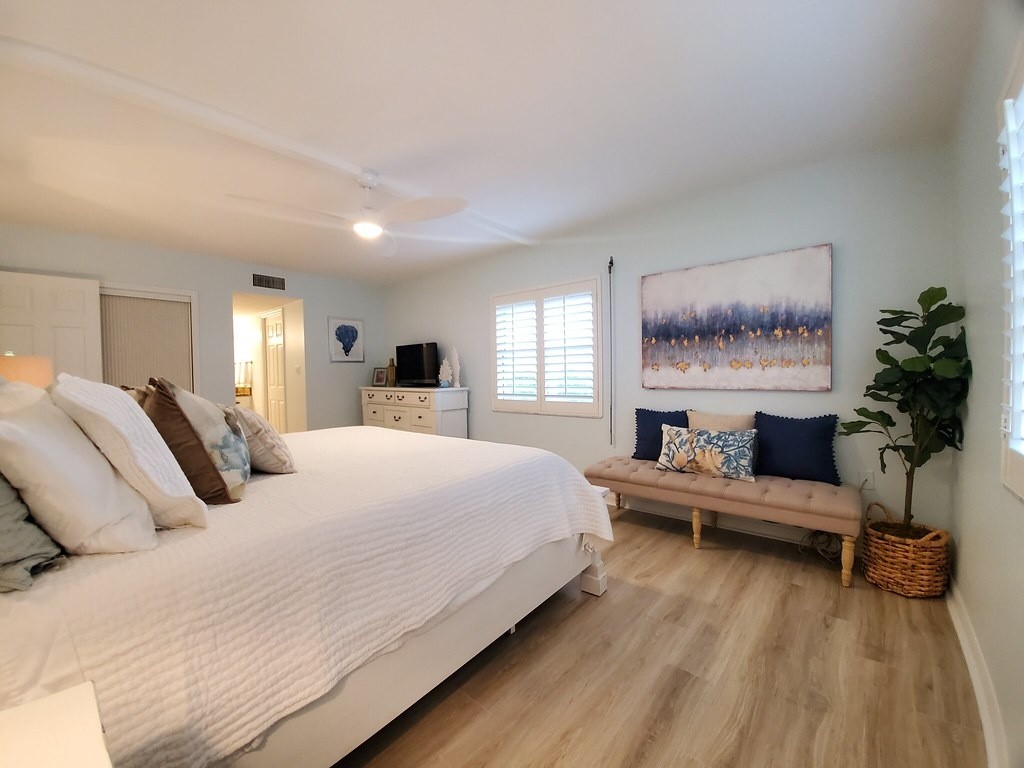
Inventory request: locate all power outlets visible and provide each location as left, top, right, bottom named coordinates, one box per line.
left=858, top=471, right=873, bottom=489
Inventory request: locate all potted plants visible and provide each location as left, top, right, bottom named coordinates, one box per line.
left=838, top=285, right=973, bottom=597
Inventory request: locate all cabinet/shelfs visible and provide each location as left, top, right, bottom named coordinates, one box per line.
left=359, top=386, right=469, bottom=438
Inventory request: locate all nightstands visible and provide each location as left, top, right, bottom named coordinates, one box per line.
left=0, top=680, right=112, bottom=768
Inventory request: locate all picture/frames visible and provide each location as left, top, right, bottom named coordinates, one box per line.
left=372, top=367, right=387, bottom=387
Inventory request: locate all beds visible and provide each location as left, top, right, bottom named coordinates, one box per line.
left=0, top=426, right=615, bottom=768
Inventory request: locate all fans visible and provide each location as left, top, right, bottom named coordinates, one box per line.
left=292, top=176, right=468, bottom=247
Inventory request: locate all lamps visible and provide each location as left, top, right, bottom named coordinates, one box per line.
left=0, top=350, right=53, bottom=388
left=351, top=221, right=383, bottom=240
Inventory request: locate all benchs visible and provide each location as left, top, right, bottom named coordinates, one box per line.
left=584, top=455, right=863, bottom=587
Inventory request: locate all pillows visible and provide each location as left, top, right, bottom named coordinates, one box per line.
left=750, top=412, right=842, bottom=487
left=631, top=407, right=689, bottom=461
left=686, top=410, right=755, bottom=431
left=655, top=423, right=758, bottom=482
left=0, top=373, right=297, bottom=593
